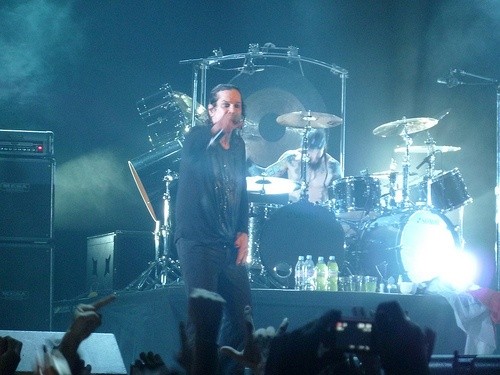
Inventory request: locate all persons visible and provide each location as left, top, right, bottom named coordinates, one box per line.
left=246, top=129, right=343, bottom=205
left=0, top=288, right=436, bottom=375
left=172, top=83, right=254, bottom=353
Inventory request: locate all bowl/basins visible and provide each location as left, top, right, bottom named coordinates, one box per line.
left=400, top=281, right=416, bottom=296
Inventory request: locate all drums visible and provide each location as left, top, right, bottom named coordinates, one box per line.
left=258, top=199, right=344, bottom=288
left=356, top=206, right=461, bottom=285
left=129, top=139, right=185, bottom=224
left=332, top=176, right=382, bottom=220
left=408, top=168, right=472, bottom=212
left=136, top=83, right=186, bottom=149
left=245, top=203, right=284, bottom=267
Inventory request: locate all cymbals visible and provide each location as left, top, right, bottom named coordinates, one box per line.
left=373, top=117, right=438, bottom=137
left=374, top=170, right=418, bottom=177
left=174, top=92, right=209, bottom=126
left=393, top=145, right=461, bottom=153
left=246, top=176, right=302, bottom=195
left=276, top=111, right=344, bottom=129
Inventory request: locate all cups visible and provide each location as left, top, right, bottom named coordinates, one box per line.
left=339, top=274, right=376, bottom=293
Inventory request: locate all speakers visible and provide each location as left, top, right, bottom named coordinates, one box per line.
left=0, top=155, right=54, bottom=328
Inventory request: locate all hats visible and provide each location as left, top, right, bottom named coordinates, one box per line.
left=306, top=129, right=327, bottom=149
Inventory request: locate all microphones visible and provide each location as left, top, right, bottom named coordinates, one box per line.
left=208, top=112, right=241, bottom=148
left=417, top=150, right=435, bottom=168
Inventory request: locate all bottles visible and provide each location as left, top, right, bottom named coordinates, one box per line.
left=295, top=256, right=306, bottom=291
left=326, top=256, right=339, bottom=292
left=314, top=256, right=329, bottom=291
left=304, top=255, right=316, bottom=292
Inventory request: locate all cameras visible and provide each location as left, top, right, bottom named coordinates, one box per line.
left=332, top=319, right=372, bottom=351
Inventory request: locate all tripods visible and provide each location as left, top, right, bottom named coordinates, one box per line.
left=123, top=168, right=188, bottom=293
